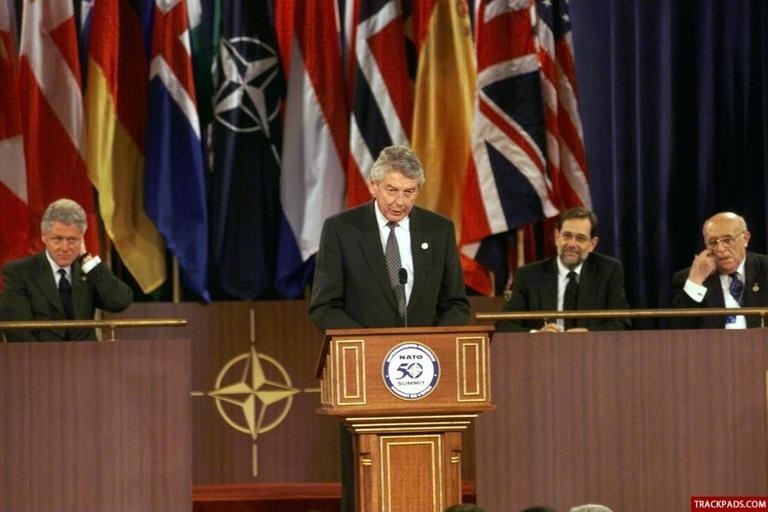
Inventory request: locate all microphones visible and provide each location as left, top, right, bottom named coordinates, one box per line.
left=398, top=268, right=408, bottom=326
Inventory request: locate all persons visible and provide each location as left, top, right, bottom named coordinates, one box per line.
left=493, top=205, right=634, bottom=334
left=664, top=210, right=768, bottom=329
left=1, top=198, right=135, bottom=341
left=307, top=145, right=471, bottom=512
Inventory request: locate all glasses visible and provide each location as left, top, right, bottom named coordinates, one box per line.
left=703, top=231, right=746, bottom=247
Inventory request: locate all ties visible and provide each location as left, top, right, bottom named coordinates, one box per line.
left=726, top=272, right=746, bottom=308
left=57, top=268, right=74, bottom=341
left=563, top=271, right=582, bottom=331
left=384, top=221, right=406, bottom=318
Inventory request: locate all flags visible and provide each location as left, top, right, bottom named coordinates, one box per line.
left=1, top=0, right=594, bottom=306
